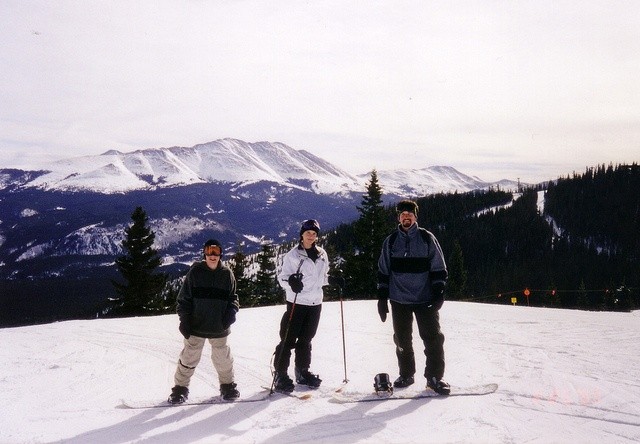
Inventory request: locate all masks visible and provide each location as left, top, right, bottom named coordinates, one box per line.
left=396, top=201, right=419, bottom=216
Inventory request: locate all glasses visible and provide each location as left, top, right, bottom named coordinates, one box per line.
left=204, top=245, right=222, bottom=255
left=301, top=219, right=320, bottom=228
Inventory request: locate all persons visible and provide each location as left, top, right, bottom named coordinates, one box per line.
left=376, top=200, right=452, bottom=394
left=271, top=219, right=330, bottom=392
left=167, top=240, right=241, bottom=404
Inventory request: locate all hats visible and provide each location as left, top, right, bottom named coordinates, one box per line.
left=300, top=219, right=320, bottom=236
left=205, top=240, right=219, bottom=245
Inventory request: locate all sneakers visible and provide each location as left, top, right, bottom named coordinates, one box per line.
left=223, top=307, right=237, bottom=329
left=296, top=371, right=322, bottom=387
left=394, top=375, right=414, bottom=387
left=220, top=382, right=239, bottom=399
left=274, top=377, right=294, bottom=392
left=427, top=378, right=450, bottom=395
left=168, top=385, right=189, bottom=404
left=179, top=322, right=191, bottom=339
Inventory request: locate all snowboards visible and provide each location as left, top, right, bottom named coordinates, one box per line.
left=332, top=373, right=498, bottom=402
left=121, top=392, right=270, bottom=409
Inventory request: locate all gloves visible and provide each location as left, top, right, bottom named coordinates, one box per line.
left=289, top=274, right=304, bottom=293
left=425, top=282, right=445, bottom=311
left=378, top=299, right=389, bottom=322
